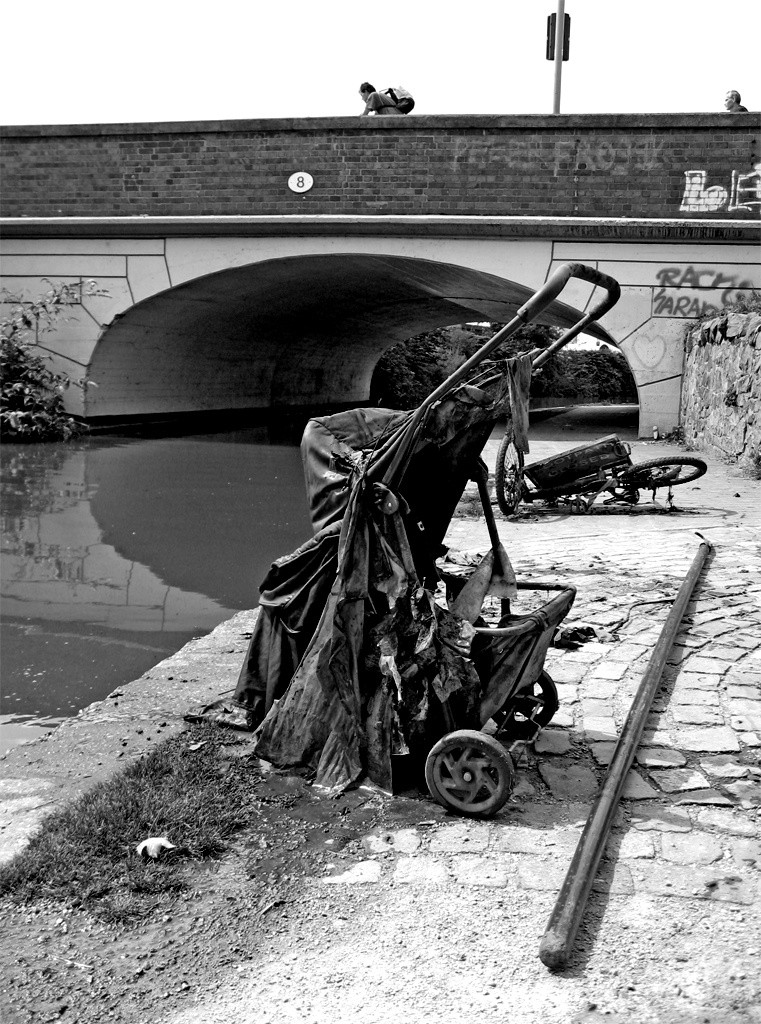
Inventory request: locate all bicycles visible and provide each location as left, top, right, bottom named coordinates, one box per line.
left=495, top=428, right=708, bottom=518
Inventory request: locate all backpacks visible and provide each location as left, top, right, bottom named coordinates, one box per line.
left=377, top=86, right=415, bottom=115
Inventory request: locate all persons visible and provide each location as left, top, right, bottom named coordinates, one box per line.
left=359, top=82, right=403, bottom=116
left=724, top=91, right=749, bottom=112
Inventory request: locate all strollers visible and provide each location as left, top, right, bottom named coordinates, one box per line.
left=234, top=261, right=623, bottom=821
